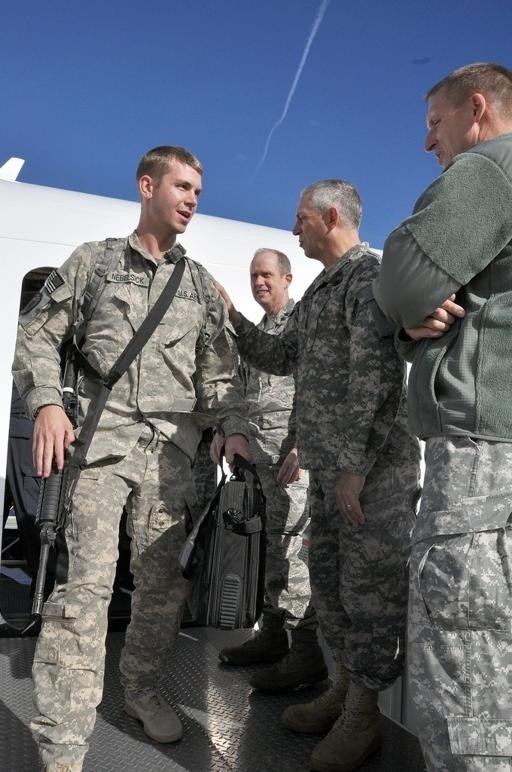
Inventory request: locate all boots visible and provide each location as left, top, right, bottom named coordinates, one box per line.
left=305, top=675, right=383, bottom=772
left=280, top=675, right=352, bottom=735
left=122, top=682, right=184, bottom=744
left=217, top=611, right=289, bottom=667
left=248, top=626, right=330, bottom=694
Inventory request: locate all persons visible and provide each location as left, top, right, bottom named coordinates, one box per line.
left=1, top=62, right=512, bottom=772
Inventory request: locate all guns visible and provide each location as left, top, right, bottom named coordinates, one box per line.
left=30, top=339, right=81, bottom=621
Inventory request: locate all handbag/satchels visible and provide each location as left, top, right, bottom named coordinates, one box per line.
left=178, top=445, right=265, bottom=631
